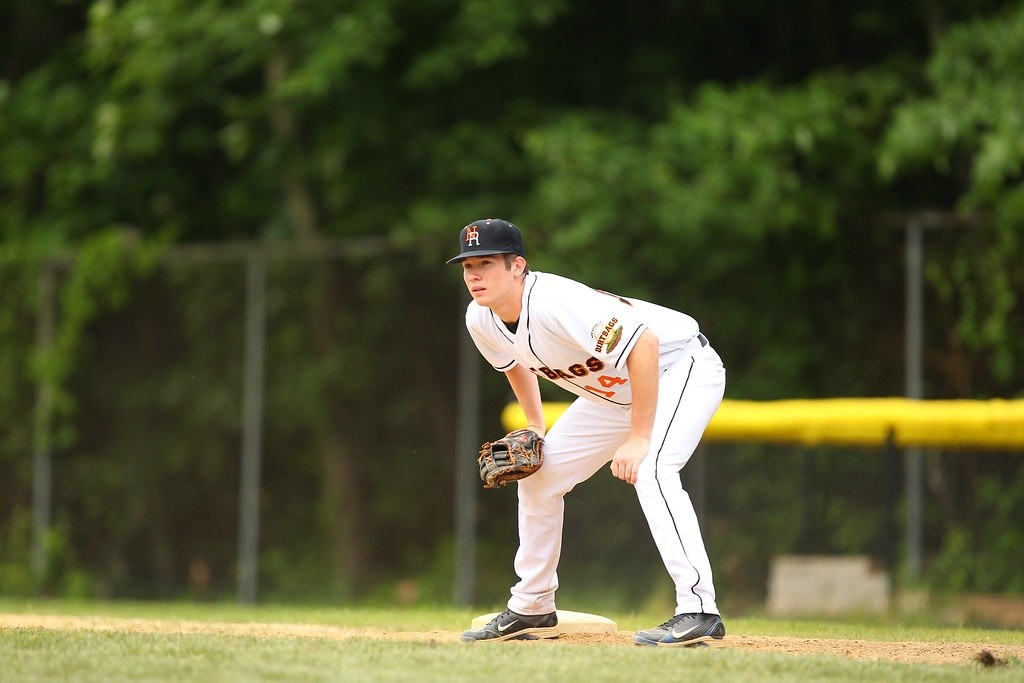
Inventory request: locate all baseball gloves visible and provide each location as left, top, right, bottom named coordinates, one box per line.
left=477, top=430, right=546, bottom=489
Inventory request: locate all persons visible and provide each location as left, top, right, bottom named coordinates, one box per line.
left=446, top=219, right=725, bottom=645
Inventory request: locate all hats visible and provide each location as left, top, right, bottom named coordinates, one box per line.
left=446, top=218, right=527, bottom=265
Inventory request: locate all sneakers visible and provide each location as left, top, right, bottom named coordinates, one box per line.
left=460, top=608, right=560, bottom=644
left=633, top=612, right=726, bottom=648
